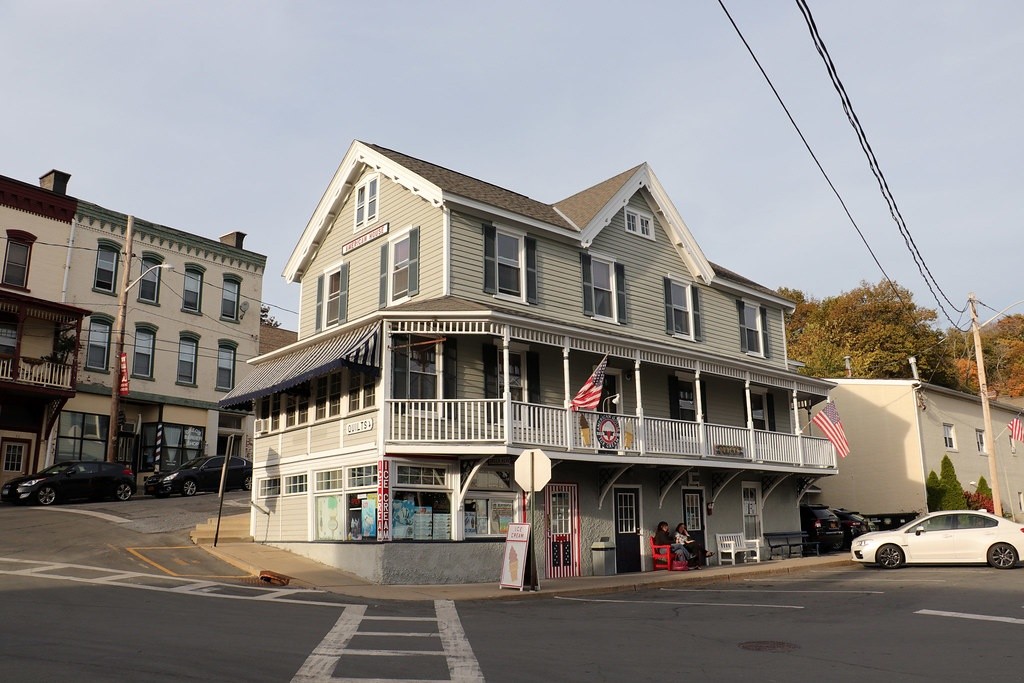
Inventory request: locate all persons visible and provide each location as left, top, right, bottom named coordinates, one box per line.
left=654, top=521, right=715, bottom=570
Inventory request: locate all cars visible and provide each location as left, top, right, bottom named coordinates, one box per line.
left=144, top=455, right=253, bottom=498
left=0, top=459, right=137, bottom=507
left=849, top=508, right=1024, bottom=570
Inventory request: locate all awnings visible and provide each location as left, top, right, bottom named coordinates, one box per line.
left=218, top=319, right=382, bottom=412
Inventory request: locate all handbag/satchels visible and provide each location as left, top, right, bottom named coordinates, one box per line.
left=671, top=553, right=688, bottom=571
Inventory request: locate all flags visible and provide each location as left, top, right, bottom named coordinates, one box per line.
left=1007, top=412, right=1024, bottom=444
left=812, top=400, right=850, bottom=458
left=571, top=356, right=607, bottom=411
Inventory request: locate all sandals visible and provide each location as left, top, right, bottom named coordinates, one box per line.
left=705, top=552, right=715, bottom=558
left=694, top=565, right=702, bottom=570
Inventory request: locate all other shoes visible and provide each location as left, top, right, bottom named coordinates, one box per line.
left=688, top=554, right=696, bottom=559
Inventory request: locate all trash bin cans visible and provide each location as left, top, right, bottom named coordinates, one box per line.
left=591, top=536, right=616, bottom=576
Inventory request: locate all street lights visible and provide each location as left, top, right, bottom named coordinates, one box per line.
left=106, top=260, right=175, bottom=464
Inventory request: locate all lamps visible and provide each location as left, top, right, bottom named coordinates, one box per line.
left=707, top=501, right=713, bottom=515
left=626, top=363, right=636, bottom=381
left=602, top=393, right=620, bottom=412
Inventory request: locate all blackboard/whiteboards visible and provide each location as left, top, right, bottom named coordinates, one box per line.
left=500, top=522, right=543, bottom=589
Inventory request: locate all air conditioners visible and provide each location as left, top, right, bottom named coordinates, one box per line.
left=121, top=422, right=136, bottom=433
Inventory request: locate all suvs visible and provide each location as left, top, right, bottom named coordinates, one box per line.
left=833, top=507, right=875, bottom=550
left=799, top=503, right=844, bottom=555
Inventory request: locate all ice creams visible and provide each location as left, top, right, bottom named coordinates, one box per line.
left=509, top=545, right=518, bottom=581
left=624, top=419, right=634, bottom=445
left=580, top=415, right=590, bottom=445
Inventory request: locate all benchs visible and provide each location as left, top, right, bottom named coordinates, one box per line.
left=716, top=533, right=761, bottom=566
left=650, top=536, right=677, bottom=571
left=763, top=531, right=820, bottom=560
